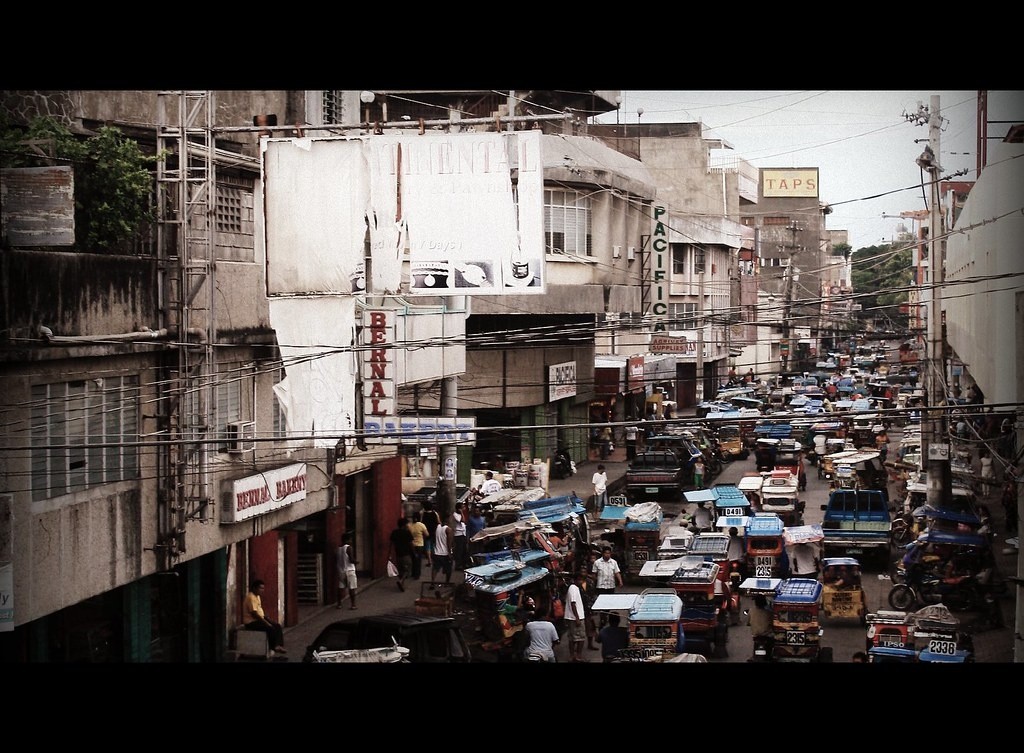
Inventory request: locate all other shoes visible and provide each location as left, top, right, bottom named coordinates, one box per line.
left=275, top=645, right=288, bottom=654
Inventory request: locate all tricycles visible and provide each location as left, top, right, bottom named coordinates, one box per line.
left=300, top=339, right=1020, bottom=663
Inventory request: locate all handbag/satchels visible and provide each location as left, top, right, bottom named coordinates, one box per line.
left=387, top=561, right=399, bottom=578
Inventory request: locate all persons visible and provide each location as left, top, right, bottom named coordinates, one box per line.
left=588, top=546, right=624, bottom=594
left=453, top=502, right=503, bottom=570
left=826, top=566, right=861, bottom=585
left=481, top=471, right=502, bottom=494
left=523, top=607, right=561, bottom=662
left=563, top=565, right=600, bottom=663
left=727, top=527, right=748, bottom=564
left=853, top=651, right=866, bottom=665
left=821, top=383, right=838, bottom=392
left=1000, top=480, right=1018, bottom=536
left=916, top=517, right=927, bottom=540
left=823, top=394, right=833, bottom=412
left=592, top=413, right=612, bottom=460
left=388, top=500, right=452, bottom=592
left=621, top=418, right=639, bottom=460
left=965, top=386, right=972, bottom=403
left=956, top=506, right=991, bottom=535
left=244, top=581, right=288, bottom=654
left=592, top=465, right=608, bottom=517
left=973, top=410, right=996, bottom=459
left=876, top=430, right=891, bottom=464
left=956, top=420, right=966, bottom=433
left=596, top=611, right=630, bottom=661
left=952, top=407, right=962, bottom=421
left=549, top=534, right=567, bottom=548
left=728, top=366, right=736, bottom=380
left=691, top=457, right=706, bottom=487
left=749, top=492, right=759, bottom=517
left=496, top=589, right=534, bottom=625
left=749, top=596, right=773, bottom=637
left=747, top=368, right=754, bottom=380
left=337, top=534, right=360, bottom=609
left=691, top=501, right=714, bottom=533
left=980, top=449, right=996, bottom=496
